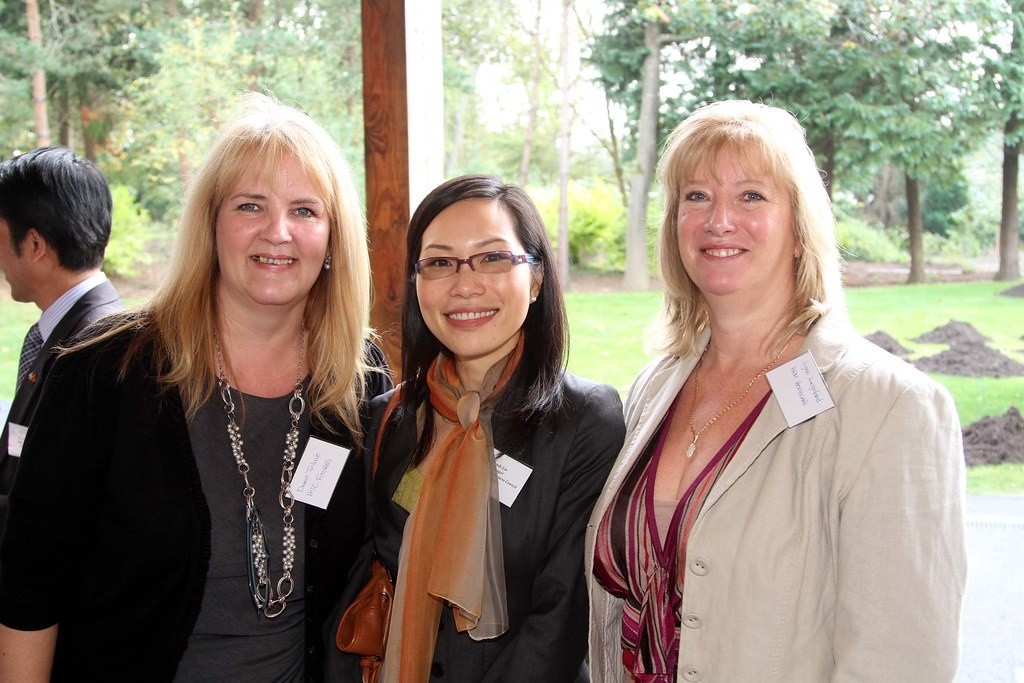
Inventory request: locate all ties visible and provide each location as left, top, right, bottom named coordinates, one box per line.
left=17, top=320, right=41, bottom=397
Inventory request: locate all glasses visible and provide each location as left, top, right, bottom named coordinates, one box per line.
left=414, top=250, right=537, bottom=280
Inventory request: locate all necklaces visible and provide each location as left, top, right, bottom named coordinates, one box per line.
left=210, top=314, right=306, bottom=617
left=687, top=324, right=802, bottom=458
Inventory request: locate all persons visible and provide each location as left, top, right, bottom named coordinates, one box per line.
left=0, top=147, right=129, bottom=543
left=585, top=100, right=968, bottom=683
left=329, top=174, right=629, bottom=683
left=0, top=91, right=394, bottom=683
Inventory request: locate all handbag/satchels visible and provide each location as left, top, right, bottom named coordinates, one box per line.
left=335, top=382, right=409, bottom=683
